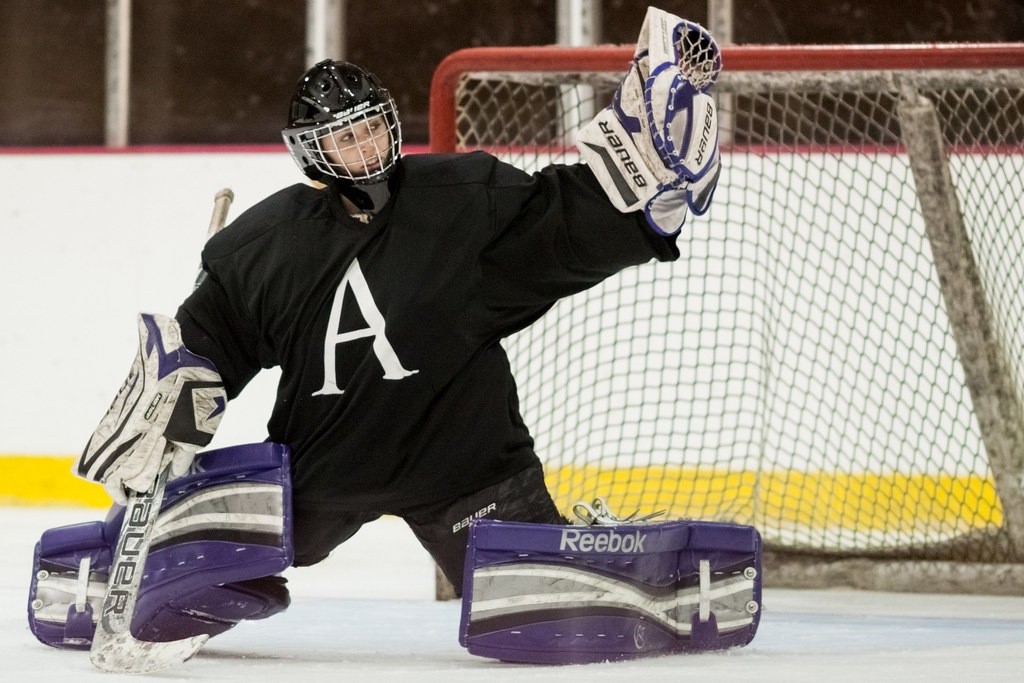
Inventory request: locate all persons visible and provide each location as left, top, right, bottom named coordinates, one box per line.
left=27, top=6, right=763, bottom=665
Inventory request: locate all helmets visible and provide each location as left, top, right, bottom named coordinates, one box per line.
left=281, top=58, right=401, bottom=210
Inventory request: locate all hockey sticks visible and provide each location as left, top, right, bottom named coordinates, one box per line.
left=90, top=188, right=236, bottom=674
